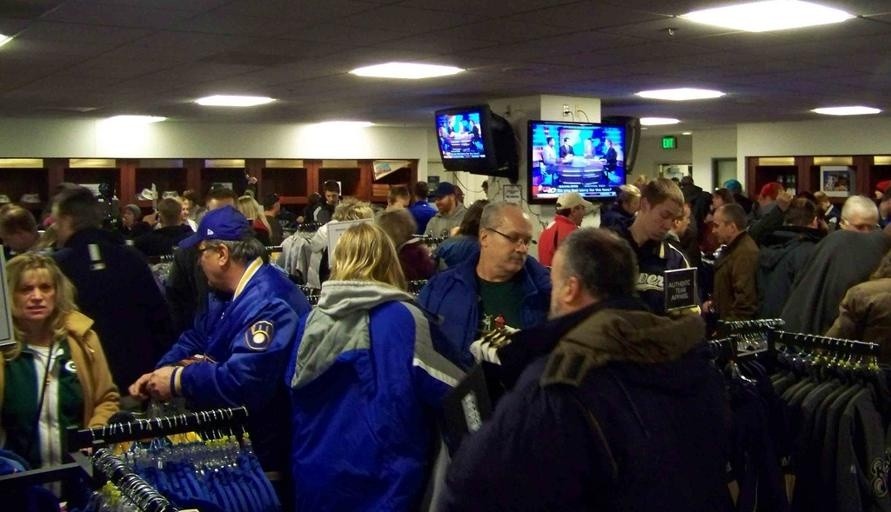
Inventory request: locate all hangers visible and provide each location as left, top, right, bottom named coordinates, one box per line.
left=83, top=404, right=257, bottom=511
left=159, top=254, right=175, bottom=264
left=296, top=278, right=322, bottom=305
left=299, top=221, right=323, bottom=235
left=423, top=228, right=449, bottom=246
left=707, top=317, right=880, bottom=386
left=408, top=278, right=428, bottom=296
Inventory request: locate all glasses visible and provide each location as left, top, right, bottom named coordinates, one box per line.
left=196, top=244, right=220, bottom=256
left=484, top=226, right=537, bottom=247
left=841, top=215, right=876, bottom=231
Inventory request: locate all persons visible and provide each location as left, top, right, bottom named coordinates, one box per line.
left=437, top=118, right=480, bottom=153
left=541, top=132, right=617, bottom=186
left=0, top=174, right=891, bottom=511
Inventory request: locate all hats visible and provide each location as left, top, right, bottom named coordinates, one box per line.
left=761, top=182, right=784, bottom=200
left=876, top=179, right=891, bottom=194
left=556, top=191, right=593, bottom=211
left=722, top=179, right=742, bottom=192
left=123, top=204, right=142, bottom=218
left=177, top=205, right=255, bottom=248
left=431, top=181, right=454, bottom=198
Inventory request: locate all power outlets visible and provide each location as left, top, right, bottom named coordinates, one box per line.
left=563, top=104, right=570, bottom=117
left=505, top=104, right=511, bottom=117
left=575, top=104, right=584, bottom=119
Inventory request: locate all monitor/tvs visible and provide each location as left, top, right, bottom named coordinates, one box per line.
left=525, top=120, right=628, bottom=205
left=434, top=103, right=518, bottom=184
left=602, top=114, right=641, bottom=174
